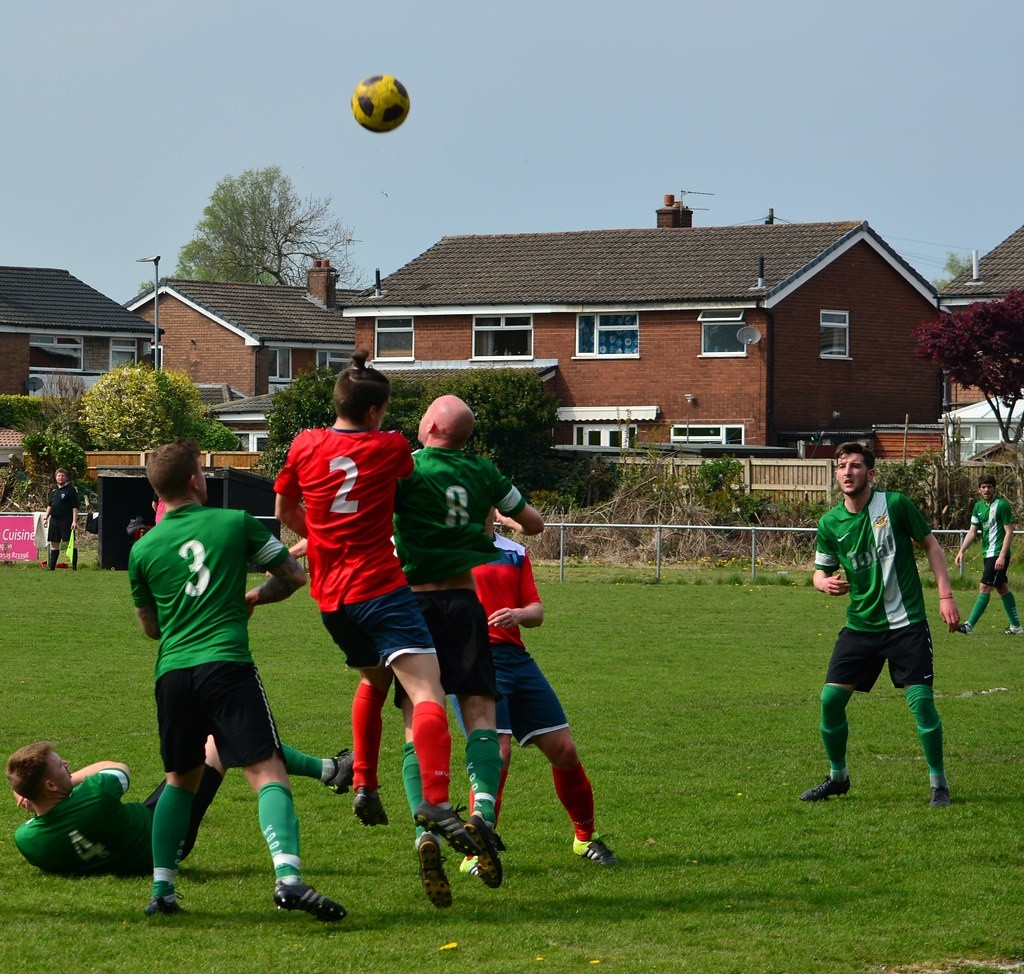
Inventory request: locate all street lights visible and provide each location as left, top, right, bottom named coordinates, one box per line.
left=136, top=254, right=163, bottom=370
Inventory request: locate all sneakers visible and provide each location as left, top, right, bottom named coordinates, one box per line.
left=144, top=893, right=190, bottom=916
left=351, top=784, right=389, bottom=827
left=413, top=802, right=482, bottom=857
left=931, top=789, right=950, bottom=807
left=800, top=776, right=851, bottom=803
left=1004, top=625, right=1023, bottom=636
left=955, top=624, right=973, bottom=636
left=573, top=830, right=617, bottom=867
left=416, top=829, right=453, bottom=910
left=459, top=855, right=481, bottom=878
left=323, top=747, right=354, bottom=793
left=466, top=813, right=503, bottom=889
left=273, top=879, right=348, bottom=922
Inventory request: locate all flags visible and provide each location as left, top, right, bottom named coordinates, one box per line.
left=66, top=529, right=75, bottom=564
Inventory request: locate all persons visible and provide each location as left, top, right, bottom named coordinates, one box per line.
left=44, top=468, right=80, bottom=571
left=799, top=442, right=961, bottom=808
left=126, top=444, right=347, bottom=921
left=269, top=350, right=613, bottom=908
left=6, top=735, right=355, bottom=877
left=955, top=475, right=1023, bottom=635
left=152, top=491, right=166, bottom=524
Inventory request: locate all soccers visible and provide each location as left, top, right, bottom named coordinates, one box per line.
left=351, top=74, right=410, bottom=133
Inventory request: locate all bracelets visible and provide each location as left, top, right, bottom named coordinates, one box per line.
left=940, top=596, right=953, bottom=599
left=44, top=518, right=47, bottom=520
